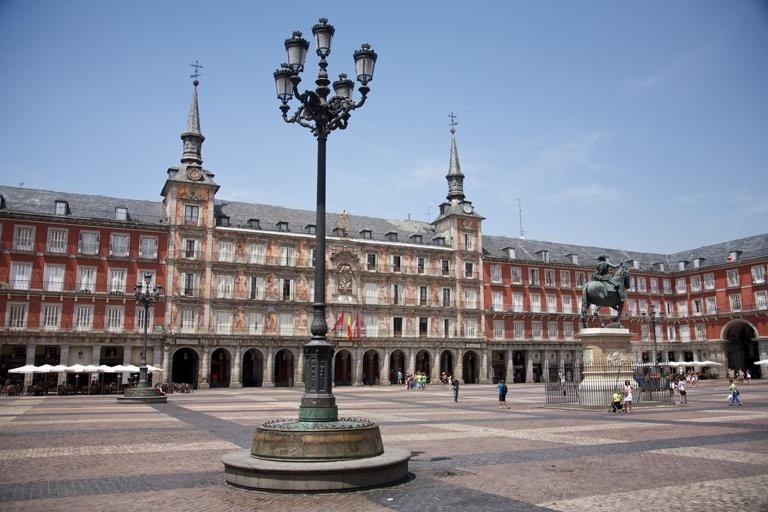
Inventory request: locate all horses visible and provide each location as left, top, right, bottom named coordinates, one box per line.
left=580, top=266, right=631, bottom=328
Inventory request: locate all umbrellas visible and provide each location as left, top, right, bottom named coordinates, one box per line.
left=754, top=359, right=768, bottom=365
left=631, top=360, right=724, bottom=375
left=8, top=364, right=165, bottom=395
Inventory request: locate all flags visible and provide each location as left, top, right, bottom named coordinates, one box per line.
left=332, top=309, right=345, bottom=331
left=356, top=311, right=361, bottom=339
left=347, top=314, right=352, bottom=340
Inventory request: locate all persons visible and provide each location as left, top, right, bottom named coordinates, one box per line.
left=683, top=371, right=699, bottom=388
left=447, top=373, right=452, bottom=390
left=668, top=378, right=677, bottom=402
left=560, top=376, right=567, bottom=396
left=451, top=377, right=460, bottom=401
left=610, top=391, right=624, bottom=412
left=677, top=376, right=687, bottom=404
left=391, top=367, right=430, bottom=390
left=728, top=380, right=743, bottom=407
left=621, top=380, right=635, bottom=414
left=593, top=254, right=627, bottom=302
left=727, top=368, right=751, bottom=384
left=440, top=372, right=447, bottom=384
left=496, top=378, right=508, bottom=409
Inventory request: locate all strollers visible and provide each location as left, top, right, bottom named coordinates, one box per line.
left=606, top=392, right=627, bottom=415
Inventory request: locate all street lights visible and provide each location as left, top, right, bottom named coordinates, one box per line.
left=272, top=8, right=381, bottom=422
left=133, top=271, right=164, bottom=388
left=641, top=304, right=665, bottom=377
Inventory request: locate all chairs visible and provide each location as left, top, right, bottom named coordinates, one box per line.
left=0, top=382, right=195, bottom=397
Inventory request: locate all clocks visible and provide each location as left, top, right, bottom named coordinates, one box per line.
left=187, top=167, right=202, bottom=182
left=463, top=203, right=473, bottom=214
